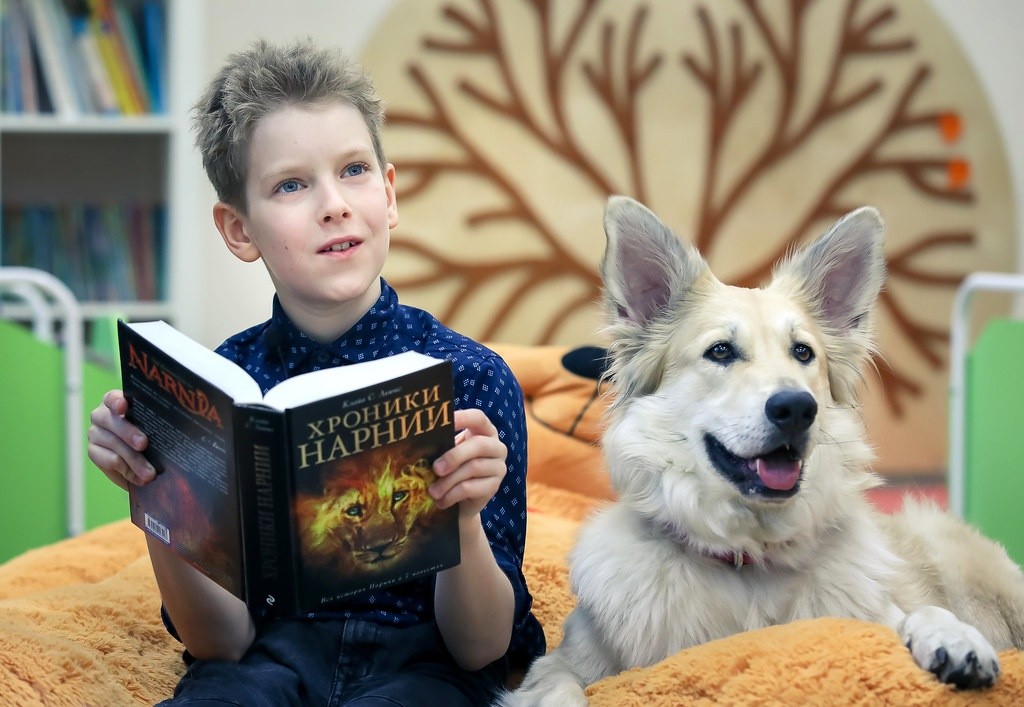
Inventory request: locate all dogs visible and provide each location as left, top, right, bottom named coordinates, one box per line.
left=491, top=194, right=1024, bottom=707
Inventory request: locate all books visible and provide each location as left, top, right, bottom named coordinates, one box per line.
left=1, top=0, right=164, bottom=302
left=118, top=319, right=461, bottom=615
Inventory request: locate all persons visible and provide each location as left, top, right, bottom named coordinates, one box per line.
left=88, top=38, right=548, bottom=706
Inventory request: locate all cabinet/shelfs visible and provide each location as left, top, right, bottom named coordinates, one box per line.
left=0, top=0, right=189, bottom=345
